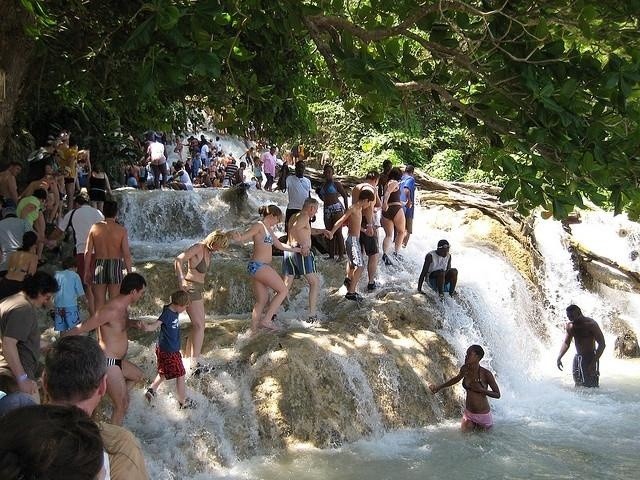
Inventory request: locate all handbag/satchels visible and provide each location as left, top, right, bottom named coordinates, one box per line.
left=56, top=209, right=76, bottom=259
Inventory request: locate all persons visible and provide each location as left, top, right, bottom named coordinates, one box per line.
left=174, top=229, right=229, bottom=358
left=225, top=146, right=414, bottom=329
left=418, top=240, right=458, bottom=298
left=145, top=131, right=225, bottom=190
left=0, top=132, right=148, bottom=480
left=557, top=305, right=605, bottom=388
left=429, top=344, right=500, bottom=434
left=147, top=291, right=199, bottom=409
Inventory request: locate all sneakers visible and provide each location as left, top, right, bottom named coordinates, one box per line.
left=145, top=388, right=156, bottom=408
left=346, top=292, right=363, bottom=301
left=178, top=400, right=195, bottom=409
left=344, top=277, right=351, bottom=288
left=367, top=281, right=381, bottom=290
